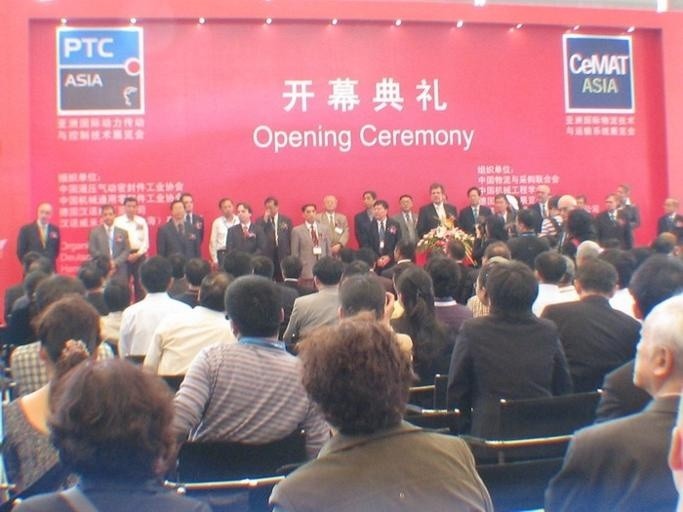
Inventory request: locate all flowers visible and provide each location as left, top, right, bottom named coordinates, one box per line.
left=413, top=216, right=477, bottom=268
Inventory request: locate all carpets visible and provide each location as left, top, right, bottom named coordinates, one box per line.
left=611, top=212, right=614, bottom=223
left=271, top=217, right=276, bottom=246
left=178, top=225, right=185, bottom=245
left=379, top=221, right=385, bottom=242
left=330, top=217, right=334, bottom=241
left=474, top=209, right=477, bottom=221
left=311, top=226, right=318, bottom=247
left=186, top=215, right=191, bottom=227
left=404, top=213, right=414, bottom=243
left=542, top=204, right=546, bottom=217
left=243, top=226, right=247, bottom=239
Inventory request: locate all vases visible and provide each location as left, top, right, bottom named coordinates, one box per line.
left=413, top=216, right=477, bottom=268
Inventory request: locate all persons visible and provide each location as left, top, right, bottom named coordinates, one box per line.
left=2, top=209, right=682, bottom=510
left=88, top=184, right=682, bottom=305
left=16, top=203, right=61, bottom=273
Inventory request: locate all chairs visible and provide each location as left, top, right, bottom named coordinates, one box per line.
left=407, top=373, right=603, bottom=509
left=178, top=426, right=305, bottom=508
